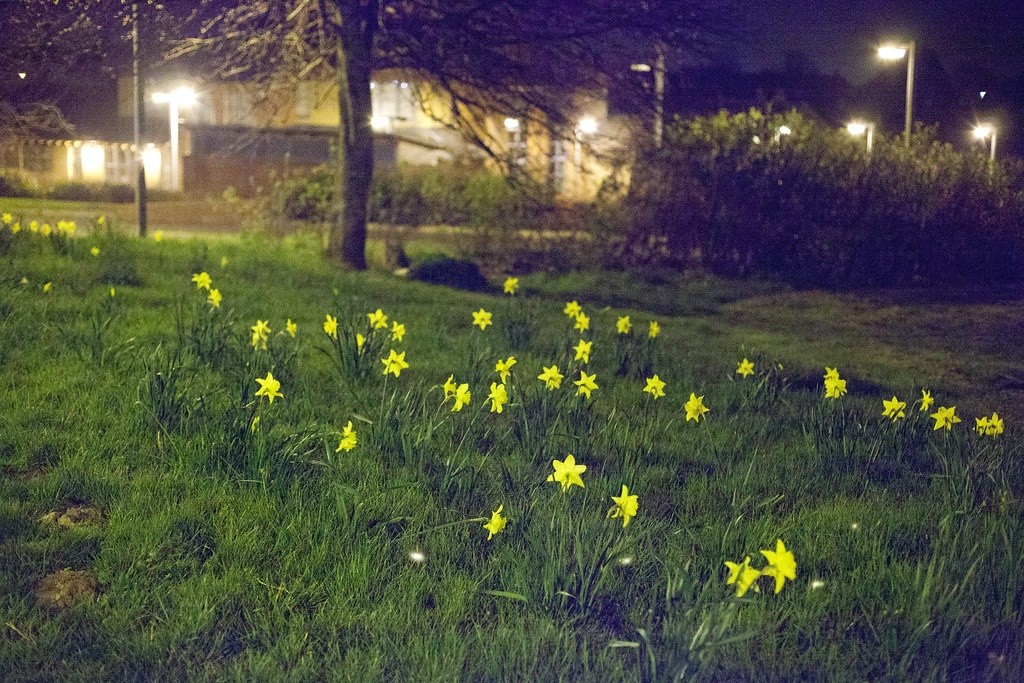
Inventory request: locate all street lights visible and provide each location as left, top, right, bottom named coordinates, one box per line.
left=876, top=39, right=915, bottom=151
left=970, top=121, right=999, bottom=188
left=631, top=55, right=666, bottom=149
